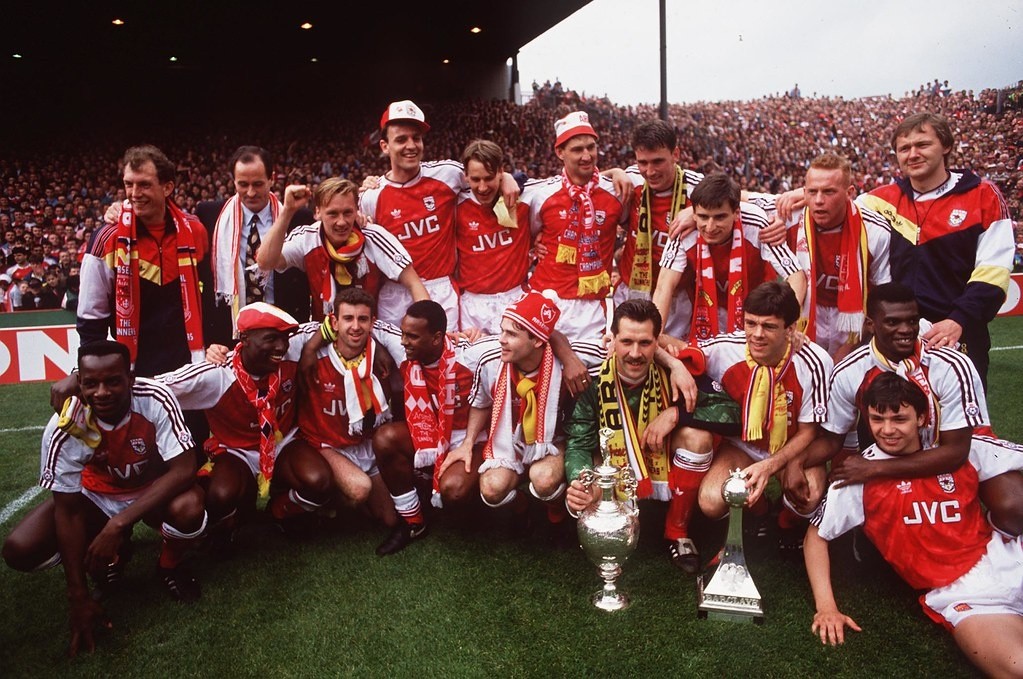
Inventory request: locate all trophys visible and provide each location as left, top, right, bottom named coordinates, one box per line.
left=577, top=429, right=640, bottom=612
left=698, top=466, right=764, bottom=624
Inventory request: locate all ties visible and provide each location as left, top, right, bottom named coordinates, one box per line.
left=245, top=214, right=266, bottom=305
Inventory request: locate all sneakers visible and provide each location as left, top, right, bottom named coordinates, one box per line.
left=853, top=525, right=882, bottom=568
left=549, top=518, right=573, bottom=548
left=375, top=520, right=430, bottom=556
left=669, top=537, right=701, bottom=573
left=774, top=525, right=805, bottom=552
left=209, top=530, right=236, bottom=551
left=266, top=501, right=309, bottom=540
left=155, top=550, right=201, bottom=603
left=743, top=495, right=778, bottom=551
left=512, top=507, right=534, bottom=538
left=92, top=553, right=130, bottom=603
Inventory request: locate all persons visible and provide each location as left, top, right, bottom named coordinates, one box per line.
left=1, top=78, right=1022, bottom=679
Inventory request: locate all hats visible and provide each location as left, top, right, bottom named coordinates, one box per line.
left=380, top=100, right=430, bottom=135
left=501, top=290, right=561, bottom=343
left=236, top=301, right=299, bottom=334
left=554, top=111, right=599, bottom=147
